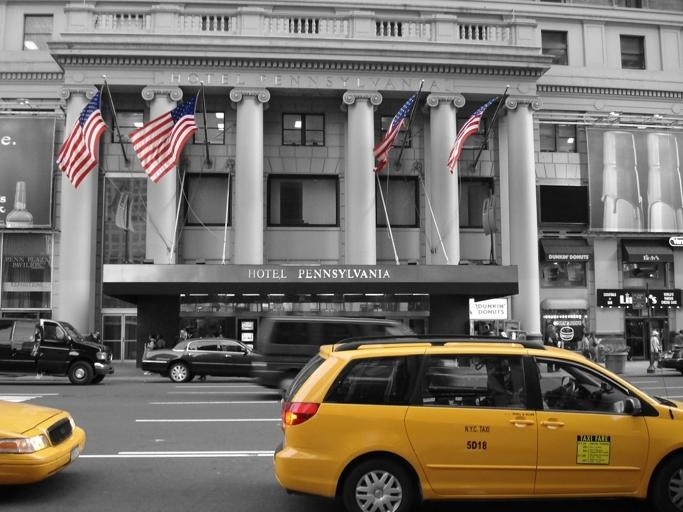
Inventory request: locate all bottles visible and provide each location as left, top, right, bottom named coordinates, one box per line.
left=4, top=181, right=33, bottom=227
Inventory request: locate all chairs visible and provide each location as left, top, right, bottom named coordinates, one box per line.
left=508, top=360, right=525, bottom=407
left=486, top=373, right=510, bottom=409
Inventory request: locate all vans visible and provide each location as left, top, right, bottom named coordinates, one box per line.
left=270, top=333, right=682, bottom=510
left=253, top=315, right=420, bottom=399
left=0, top=317, right=115, bottom=384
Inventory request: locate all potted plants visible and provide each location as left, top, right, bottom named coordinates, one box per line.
left=604, top=343, right=631, bottom=374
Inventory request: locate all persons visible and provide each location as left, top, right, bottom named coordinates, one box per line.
left=544, top=319, right=602, bottom=373
left=143, top=326, right=224, bottom=382
left=650, top=329, right=683, bottom=370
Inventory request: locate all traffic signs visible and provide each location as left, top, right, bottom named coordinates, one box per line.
left=632, top=293, right=645, bottom=301
left=633, top=302, right=646, bottom=310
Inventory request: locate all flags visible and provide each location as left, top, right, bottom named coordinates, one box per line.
left=446, top=96, right=498, bottom=175
left=372, top=92, right=418, bottom=173
left=127, top=87, right=201, bottom=185
left=55, top=86, right=109, bottom=190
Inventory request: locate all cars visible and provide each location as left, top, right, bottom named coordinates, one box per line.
left=658, top=345, right=682, bottom=373
left=0, top=394, right=89, bottom=489
left=141, top=337, right=261, bottom=383
